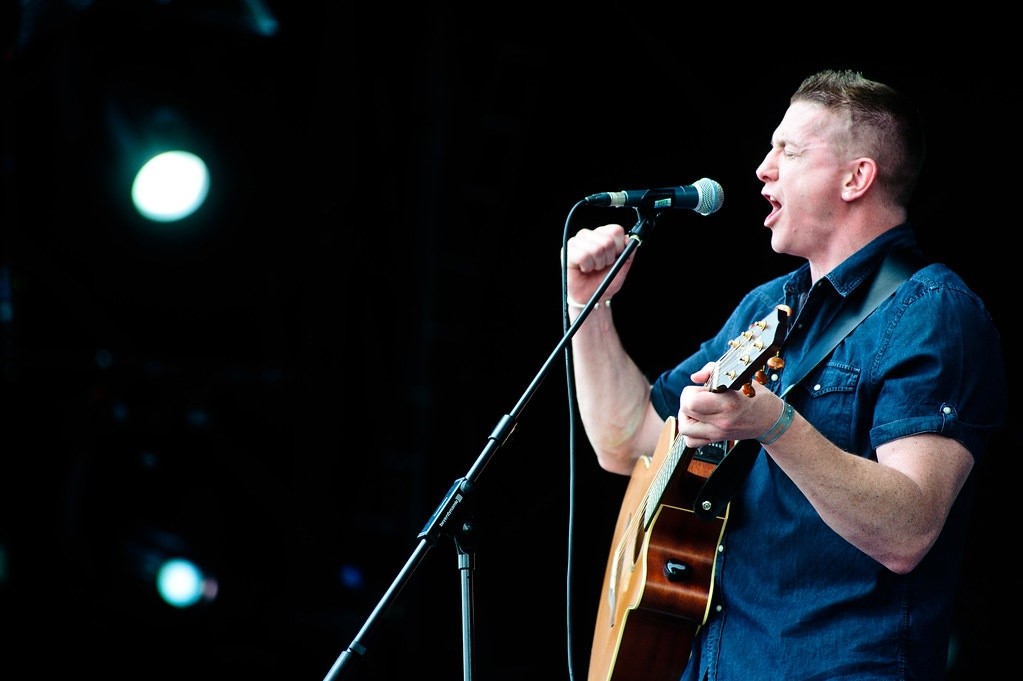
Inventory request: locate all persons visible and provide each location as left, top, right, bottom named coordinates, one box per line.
left=560, top=71, right=999, bottom=681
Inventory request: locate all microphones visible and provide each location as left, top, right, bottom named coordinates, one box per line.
left=585, top=178, right=725, bottom=216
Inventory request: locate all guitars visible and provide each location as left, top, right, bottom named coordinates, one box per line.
left=586, top=307, right=789, bottom=679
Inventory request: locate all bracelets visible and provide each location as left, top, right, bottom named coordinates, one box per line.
left=568, top=299, right=612, bottom=310
left=756, top=400, right=795, bottom=446
